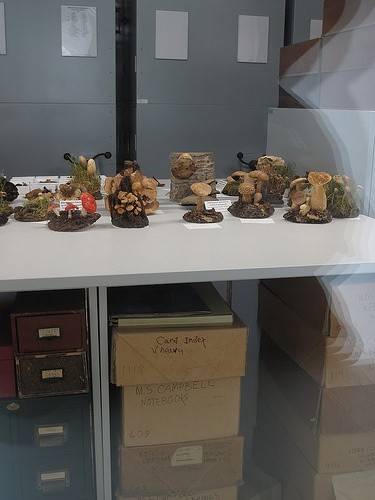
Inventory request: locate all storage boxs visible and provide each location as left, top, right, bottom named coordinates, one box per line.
left=259, top=277, right=375, bottom=338
left=110, top=377, right=241, bottom=447
left=321, top=68, right=374, bottom=110
left=254, top=391, right=374, bottom=500
left=278, top=37, right=323, bottom=79
left=14, top=351, right=91, bottom=399
left=258, top=330, right=374, bottom=434
left=112, top=432, right=282, bottom=499
left=109, top=283, right=248, bottom=388
left=0, top=397, right=97, bottom=500
left=322, top=0, right=375, bottom=38
left=278, top=73, right=322, bottom=109
left=10, top=287, right=88, bottom=355
left=258, top=359, right=374, bottom=471
left=257, top=281, right=374, bottom=387
left=0, top=336, right=17, bottom=398
left=321, top=25, right=375, bottom=74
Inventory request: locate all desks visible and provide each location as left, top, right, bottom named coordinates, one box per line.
left=0, top=179, right=375, bottom=500
